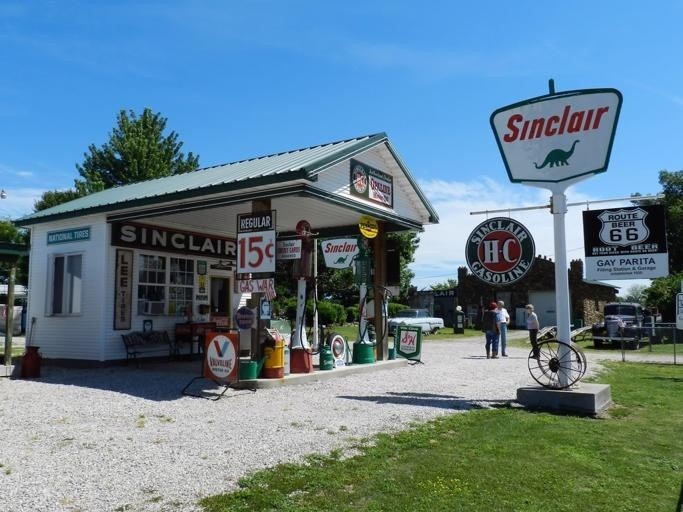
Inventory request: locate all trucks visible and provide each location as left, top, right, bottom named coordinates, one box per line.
left=592, top=302, right=663, bottom=349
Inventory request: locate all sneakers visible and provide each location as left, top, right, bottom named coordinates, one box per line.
left=529, top=353, right=540, bottom=359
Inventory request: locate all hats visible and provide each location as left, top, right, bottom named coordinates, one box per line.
left=488, top=302, right=498, bottom=308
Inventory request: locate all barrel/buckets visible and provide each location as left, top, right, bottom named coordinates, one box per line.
left=319, top=345, right=333, bottom=369
left=21, top=346, right=40, bottom=378
left=263, top=341, right=285, bottom=379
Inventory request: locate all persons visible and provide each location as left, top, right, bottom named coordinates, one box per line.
left=494, top=300, right=509, bottom=356
left=480, top=302, right=499, bottom=360
left=523, top=304, right=540, bottom=359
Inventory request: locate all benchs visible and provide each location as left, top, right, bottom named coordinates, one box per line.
left=122, top=330, right=176, bottom=369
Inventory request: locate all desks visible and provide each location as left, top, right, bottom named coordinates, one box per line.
left=175, top=322, right=217, bottom=361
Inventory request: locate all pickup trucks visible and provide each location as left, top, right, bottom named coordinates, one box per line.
left=388, top=309, right=444, bottom=335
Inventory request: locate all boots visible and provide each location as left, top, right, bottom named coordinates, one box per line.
left=486, top=349, right=509, bottom=359
